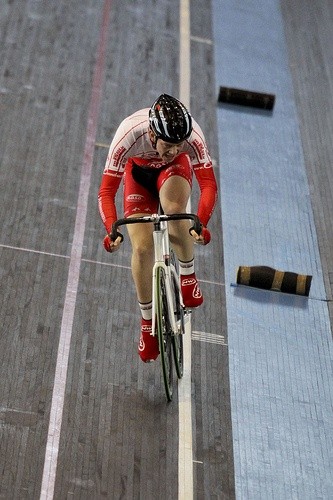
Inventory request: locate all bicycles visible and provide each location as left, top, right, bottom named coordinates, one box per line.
left=110, top=200, right=202, bottom=403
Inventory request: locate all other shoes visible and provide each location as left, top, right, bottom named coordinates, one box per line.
left=138, top=318, right=158, bottom=363
left=180, top=272, right=203, bottom=307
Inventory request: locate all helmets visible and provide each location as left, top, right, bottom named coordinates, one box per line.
left=148, top=93, right=192, bottom=143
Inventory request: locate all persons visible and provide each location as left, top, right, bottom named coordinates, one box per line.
left=98, top=93, right=218, bottom=364
